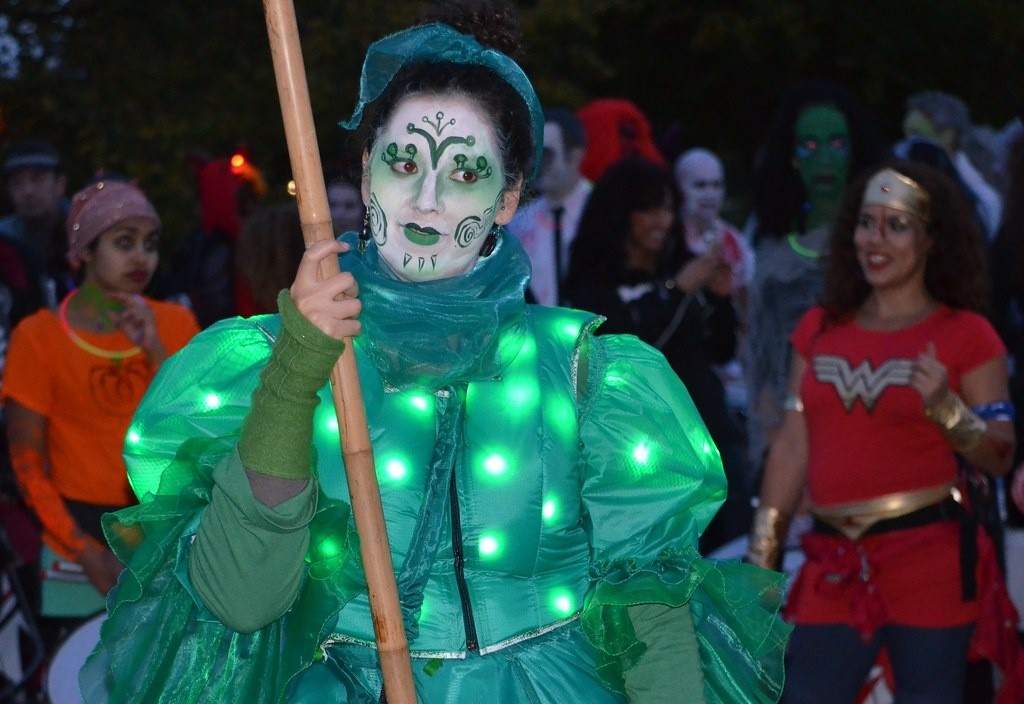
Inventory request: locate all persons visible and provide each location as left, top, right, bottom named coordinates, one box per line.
left=0, top=180, right=204, bottom=639
left=754, top=136, right=1018, bottom=704
left=124, top=17, right=753, bottom=703
left=752, top=108, right=859, bottom=472
left=0, top=126, right=83, bottom=370
left=906, top=93, right=1004, bottom=238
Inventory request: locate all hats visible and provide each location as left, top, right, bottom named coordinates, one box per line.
left=68, top=179, right=162, bottom=263
